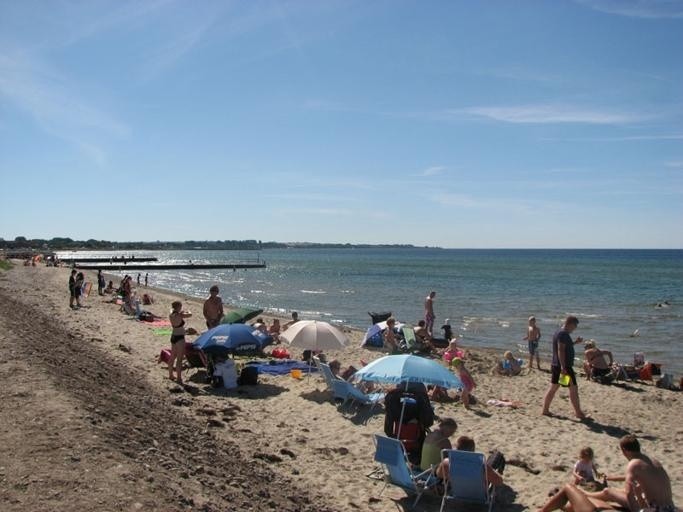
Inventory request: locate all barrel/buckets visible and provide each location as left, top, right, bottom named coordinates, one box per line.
left=558, top=372, right=570, bottom=387
left=291, top=369, right=302, bottom=378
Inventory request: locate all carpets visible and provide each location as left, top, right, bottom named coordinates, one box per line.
left=107, top=290, right=317, bottom=378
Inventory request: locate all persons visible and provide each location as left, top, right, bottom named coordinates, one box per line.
left=68, top=269, right=151, bottom=315
left=168, top=302, right=192, bottom=384
left=25, top=253, right=58, bottom=267
left=253, top=312, right=300, bottom=339
left=327, top=289, right=673, bottom=510
left=203, top=286, right=223, bottom=329
left=210, top=353, right=238, bottom=391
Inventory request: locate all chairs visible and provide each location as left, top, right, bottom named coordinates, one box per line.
left=594, top=364, right=630, bottom=384
left=329, top=378, right=422, bottom=420
left=368, top=431, right=440, bottom=512
left=316, top=356, right=339, bottom=379
left=438, top=445, right=497, bottom=510
